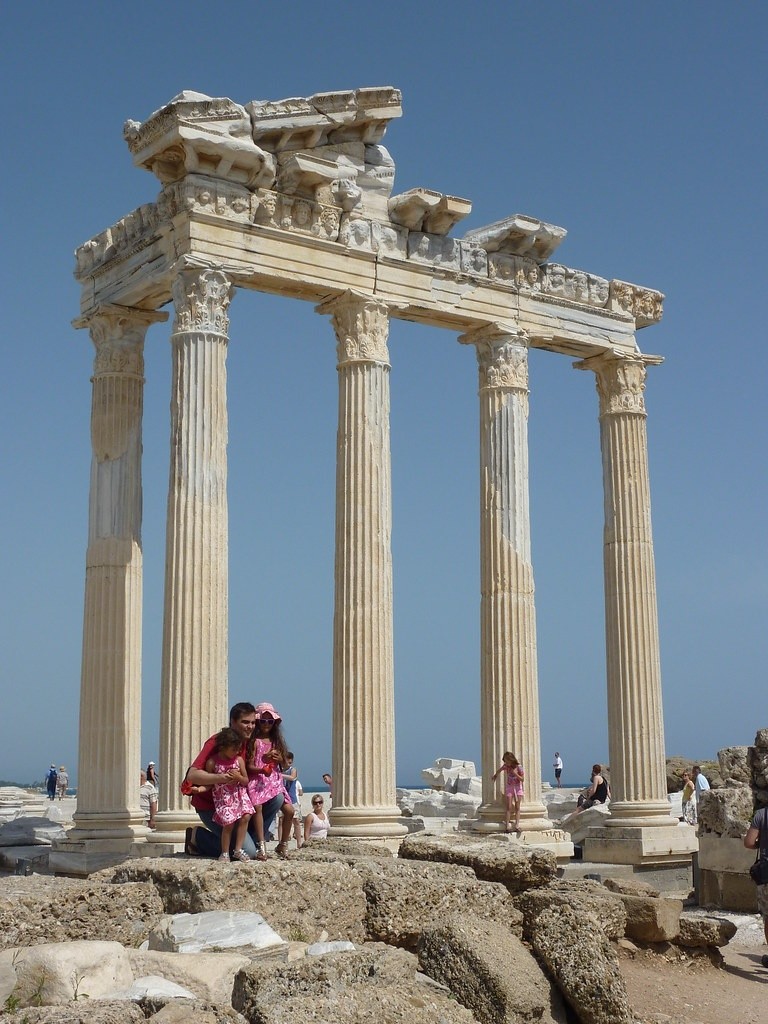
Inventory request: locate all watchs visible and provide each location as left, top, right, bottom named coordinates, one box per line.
left=151, top=816, right=154, bottom=820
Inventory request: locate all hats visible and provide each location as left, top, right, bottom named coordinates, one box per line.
left=148, top=761, right=155, bottom=765
left=50, top=764, right=55, bottom=768
left=255, top=702, right=282, bottom=721
left=60, top=766, right=66, bottom=772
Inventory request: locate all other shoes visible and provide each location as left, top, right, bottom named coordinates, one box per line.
left=504, top=829, right=510, bottom=833
left=515, top=828, right=522, bottom=832
left=557, top=785, right=563, bottom=788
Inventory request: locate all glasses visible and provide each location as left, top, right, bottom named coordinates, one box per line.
left=259, top=719, right=275, bottom=725
left=682, top=774, right=687, bottom=776
left=313, top=801, right=323, bottom=805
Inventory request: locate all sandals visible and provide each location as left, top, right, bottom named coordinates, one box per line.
left=256, top=841, right=268, bottom=861
left=233, top=849, right=250, bottom=863
left=275, top=841, right=290, bottom=860
left=218, top=853, right=230, bottom=864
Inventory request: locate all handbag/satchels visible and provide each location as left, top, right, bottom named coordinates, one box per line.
left=750, top=858, right=768, bottom=886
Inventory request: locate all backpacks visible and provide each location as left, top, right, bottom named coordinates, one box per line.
left=50, top=770, right=57, bottom=781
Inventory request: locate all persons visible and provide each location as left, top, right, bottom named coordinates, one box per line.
left=572, top=764, right=611, bottom=816
left=492, top=752, right=524, bottom=832
left=744, top=807, right=768, bottom=968
left=45, top=764, right=69, bottom=801
left=112, top=187, right=176, bottom=247
left=255, top=193, right=655, bottom=312
left=181, top=702, right=332, bottom=863
left=553, top=752, right=563, bottom=788
left=682, top=766, right=710, bottom=825
left=140, top=761, right=159, bottom=829
